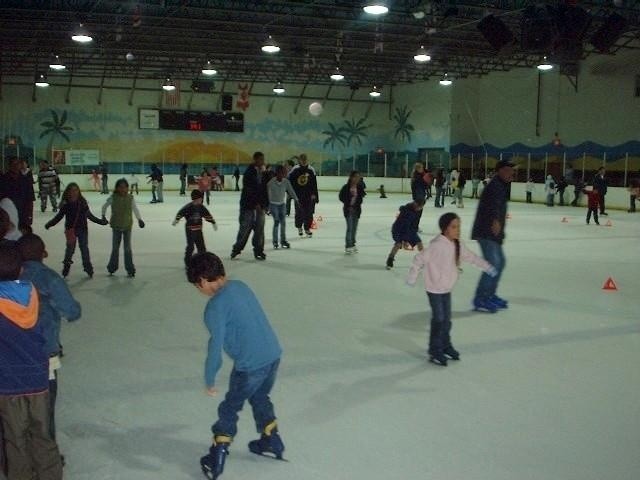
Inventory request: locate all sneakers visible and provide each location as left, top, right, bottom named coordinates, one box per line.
left=281, top=241, right=288, bottom=246
left=387, top=257, right=393, bottom=267
left=443, top=346, right=459, bottom=357
left=428, top=348, right=446, bottom=362
left=305, top=230, right=312, bottom=235
left=299, top=228, right=302, bottom=235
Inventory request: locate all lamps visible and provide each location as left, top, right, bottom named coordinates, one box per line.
left=329, top=67, right=345, bottom=80
left=273, top=81, right=284, bottom=93
left=162, top=74, right=175, bottom=91
left=35, top=74, right=50, bottom=87
left=369, top=85, right=381, bottom=97
left=202, top=60, right=217, bottom=75
left=260, top=35, right=280, bottom=53
left=439, top=73, right=452, bottom=85
left=477, top=2, right=628, bottom=71
left=49, top=56, right=66, bottom=68
left=363, top=1, right=389, bottom=14
left=71, top=22, right=92, bottom=42
left=414, top=45, right=431, bottom=62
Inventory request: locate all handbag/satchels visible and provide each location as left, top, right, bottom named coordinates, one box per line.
left=64, top=228, right=76, bottom=245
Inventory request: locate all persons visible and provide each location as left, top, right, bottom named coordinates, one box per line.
left=386, top=198, right=423, bottom=268
left=411, top=160, right=640, bottom=225
left=358, top=176, right=366, bottom=198
left=0, top=237, right=62, bottom=480
left=0, top=152, right=320, bottom=279
left=186, top=252, right=283, bottom=473
left=15, top=232, right=83, bottom=465
left=470, top=161, right=516, bottom=314
left=339, top=172, right=361, bottom=254
left=376, top=185, right=387, bottom=198
left=406, top=214, right=499, bottom=365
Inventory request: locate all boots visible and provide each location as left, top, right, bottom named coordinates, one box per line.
left=490, top=293, right=507, bottom=305
left=200, top=434, right=231, bottom=474
left=473, top=288, right=496, bottom=308
left=249, top=418, right=284, bottom=452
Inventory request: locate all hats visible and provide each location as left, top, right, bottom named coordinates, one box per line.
left=496, top=160, right=516, bottom=171
